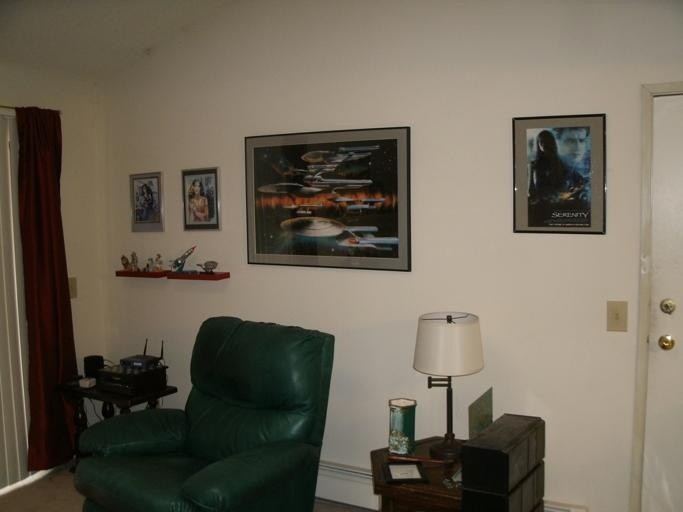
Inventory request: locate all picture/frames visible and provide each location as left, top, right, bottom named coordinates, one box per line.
left=127, top=170, right=165, bottom=234
left=179, top=165, right=221, bottom=234
left=509, top=112, right=609, bottom=237
left=241, top=123, right=414, bottom=275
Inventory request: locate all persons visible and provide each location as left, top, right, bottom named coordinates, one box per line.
left=134, top=180, right=157, bottom=221
left=186, top=178, right=212, bottom=220
left=555, top=127, right=589, bottom=168
left=527, top=130, right=585, bottom=206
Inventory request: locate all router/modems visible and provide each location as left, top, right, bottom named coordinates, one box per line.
left=120, top=337, right=163, bottom=369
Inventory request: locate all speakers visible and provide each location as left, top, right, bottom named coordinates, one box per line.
left=84, top=355, right=104, bottom=377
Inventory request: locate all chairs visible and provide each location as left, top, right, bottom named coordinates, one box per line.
left=73, top=311, right=337, bottom=511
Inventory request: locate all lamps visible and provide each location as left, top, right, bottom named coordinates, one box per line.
left=409, top=307, right=488, bottom=460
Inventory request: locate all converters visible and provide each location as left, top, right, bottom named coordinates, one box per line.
left=79, top=378, right=97, bottom=387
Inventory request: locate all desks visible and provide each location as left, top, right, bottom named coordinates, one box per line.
left=367, top=433, right=470, bottom=511
left=59, top=379, right=179, bottom=434
